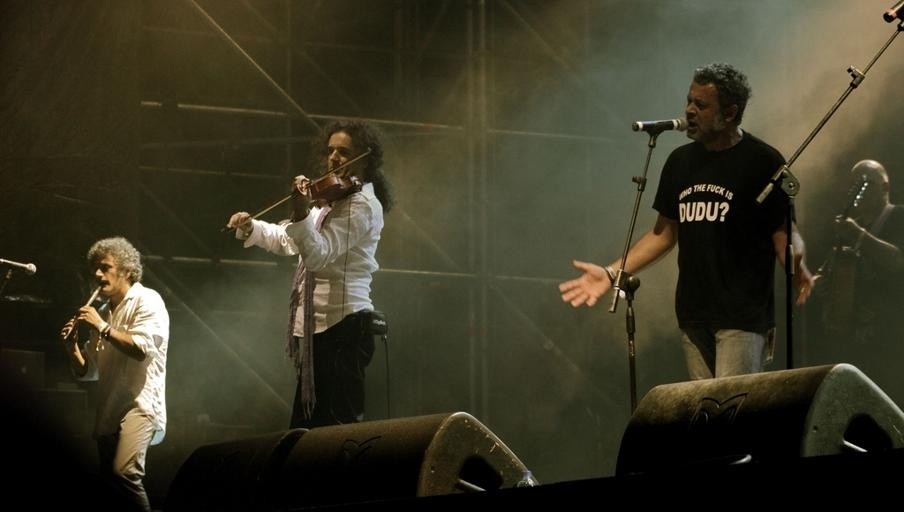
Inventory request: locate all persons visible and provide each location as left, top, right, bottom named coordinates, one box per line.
left=57, top=236, right=173, bottom=512
left=226, top=119, right=395, bottom=433
left=822, top=159, right=903, bottom=417
left=553, top=59, right=818, bottom=385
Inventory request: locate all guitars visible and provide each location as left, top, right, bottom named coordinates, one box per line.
left=812, top=175, right=872, bottom=327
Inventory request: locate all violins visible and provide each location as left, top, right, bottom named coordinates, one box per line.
left=297, top=175, right=363, bottom=203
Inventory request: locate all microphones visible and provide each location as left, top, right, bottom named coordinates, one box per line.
left=880, top=0, right=904, bottom=23
left=0, top=257, right=38, bottom=277
left=631, top=120, right=690, bottom=135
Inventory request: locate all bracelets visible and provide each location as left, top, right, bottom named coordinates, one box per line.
left=854, top=228, right=865, bottom=251
left=604, top=264, right=617, bottom=285
left=97, top=322, right=111, bottom=342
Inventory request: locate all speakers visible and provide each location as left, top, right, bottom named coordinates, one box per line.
left=172, top=427, right=307, bottom=511
left=614, top=361, right=903, bottom=479
left=281, top=410, right=541, bottom=512
